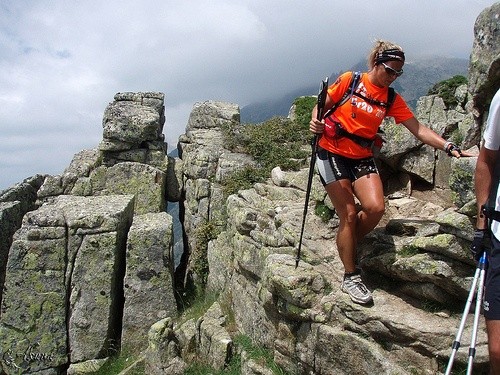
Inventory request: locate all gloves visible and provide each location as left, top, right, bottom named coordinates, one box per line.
left=471, top=229, right=490, bottom=261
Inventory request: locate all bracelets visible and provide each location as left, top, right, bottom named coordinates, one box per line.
left=443, top=141, right=455, bottom=152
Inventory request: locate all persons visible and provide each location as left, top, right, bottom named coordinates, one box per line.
left=475, top=88, right=500, bottom=375
left=309, top=41, right=479, bottom=303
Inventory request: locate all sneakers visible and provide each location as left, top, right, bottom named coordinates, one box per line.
left=341, top=275, right=372, bottom=303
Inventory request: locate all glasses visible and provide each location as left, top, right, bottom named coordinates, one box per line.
left=382, top=63, right=403, bottom=77
